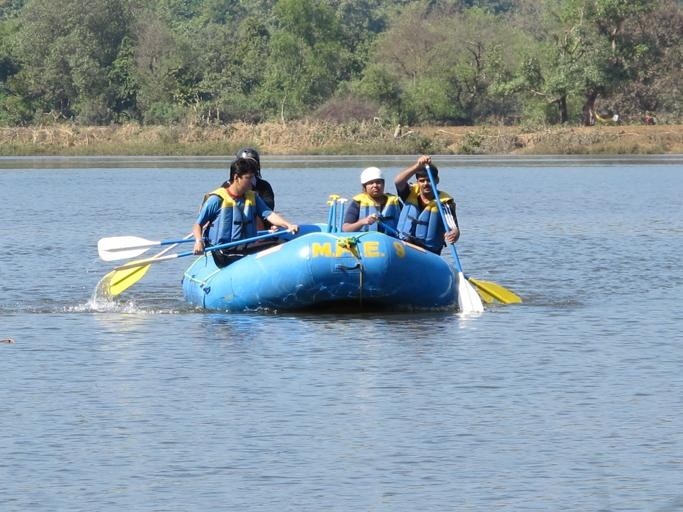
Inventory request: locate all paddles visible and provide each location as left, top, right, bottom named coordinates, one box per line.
left=94, top=220, right=292, bottom=297
left=379, top=162, right=522, bottom=317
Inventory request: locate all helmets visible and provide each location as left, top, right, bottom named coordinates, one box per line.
left=236, top=147, right=259, bottom=164
left=361, top=167, right=384, bottom=184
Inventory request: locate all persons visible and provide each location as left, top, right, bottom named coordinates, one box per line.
left=392, top=154, right=459, bottom=259
left=341, top=166, right=404, bottom=240
left=192, top=157, right=298, bottom=270
left=217, top=145, right=276, bottom=240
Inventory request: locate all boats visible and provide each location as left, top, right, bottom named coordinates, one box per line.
left=180, top=222, right=456, bottom=314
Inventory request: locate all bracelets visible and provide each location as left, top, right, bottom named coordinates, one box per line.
left=194, top=237, right=203, bottom=242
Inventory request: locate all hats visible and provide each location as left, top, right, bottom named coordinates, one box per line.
left=416, top=165, right=438, bottom=176
